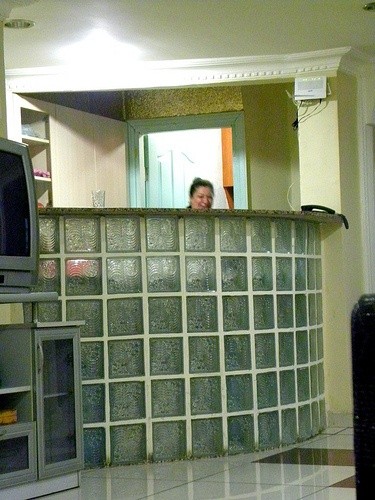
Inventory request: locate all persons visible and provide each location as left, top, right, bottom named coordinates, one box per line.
left=187, top=177, right=214, bottom=209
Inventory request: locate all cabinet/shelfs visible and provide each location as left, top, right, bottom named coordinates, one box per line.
left=7, top=94, right=128, bottom=210
left=0, top=319, right=87, bottom=500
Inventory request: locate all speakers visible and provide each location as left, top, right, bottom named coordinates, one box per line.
left=293, top=77, right=327, bottom=102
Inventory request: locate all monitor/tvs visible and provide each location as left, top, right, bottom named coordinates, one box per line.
left=0, top=137, right=39, bottom=293
